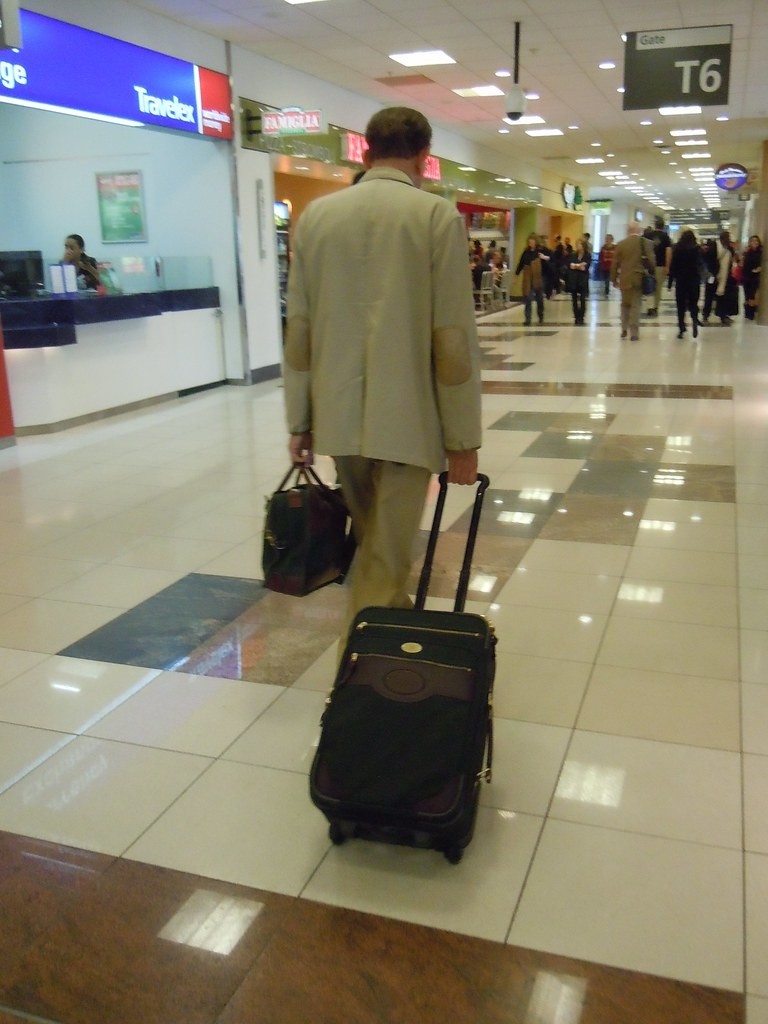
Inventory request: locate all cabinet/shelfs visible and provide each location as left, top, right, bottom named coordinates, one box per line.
left=277, top=228, right=292, bottom=320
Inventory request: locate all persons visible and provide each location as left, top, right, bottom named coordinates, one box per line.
left=641, top=220, right=672, bottom=315
left=743, top=235, right=763, bottom=319
left=703, top=232, right=740, bottom=323
left=514, top=233, right=554, bottom=326
left=61, top=234, right=100, bottom=289
left=540, top=232, right=592, bottom=300
left=284, top=107, right=482, bottom=661
left=702, top=238, right=743, bottom=285
left=599, top=234, right=617, bottom=295
left=666, top=230, right=705, bottom=339
left=566, top=239, right=592, bottom=325
left=611, top=221, right=656, bottom=340
left=469, top=239, right=510, bottom=309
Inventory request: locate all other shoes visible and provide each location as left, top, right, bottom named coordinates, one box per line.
left=524, top=319, right=530, bottom=326
left=575, top=318, right=583, bottom=324
left=677, top=326, right=686, bottom=338
left=631, top=335, right=639, bottom=341
left=538, top=315, right=545, bottom=322
left=722, top=316, right=733, bottom=324
left=621, top=330, right=627, bottom=337
left=700, top=318, right=710, bottom=327
left=693, top=325, right=698, bottom=338
left=648, top=309, right=657, bottom=316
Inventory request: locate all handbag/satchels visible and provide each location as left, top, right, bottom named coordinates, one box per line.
left=642, top=276, right=657, bottom=295
left=696, top=245, right=708, bottom=283
left=261, top=460, right=348, bottom=599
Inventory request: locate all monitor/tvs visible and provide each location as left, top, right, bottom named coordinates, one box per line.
left=0, top=251, right=44, bottom=301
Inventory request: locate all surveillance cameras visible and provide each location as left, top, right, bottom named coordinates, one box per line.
left=504, top=83, right=526, bottom=121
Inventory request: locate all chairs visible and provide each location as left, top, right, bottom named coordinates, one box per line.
left=494, top=269, right=515, bottom=305
left=474, top=269, right=497, bottom=311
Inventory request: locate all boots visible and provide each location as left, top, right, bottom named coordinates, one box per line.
left=743, top=304, right=755, bottom=320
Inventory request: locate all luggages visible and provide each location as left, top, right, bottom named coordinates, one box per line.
left=308, top=470, right=499, bottom=866
